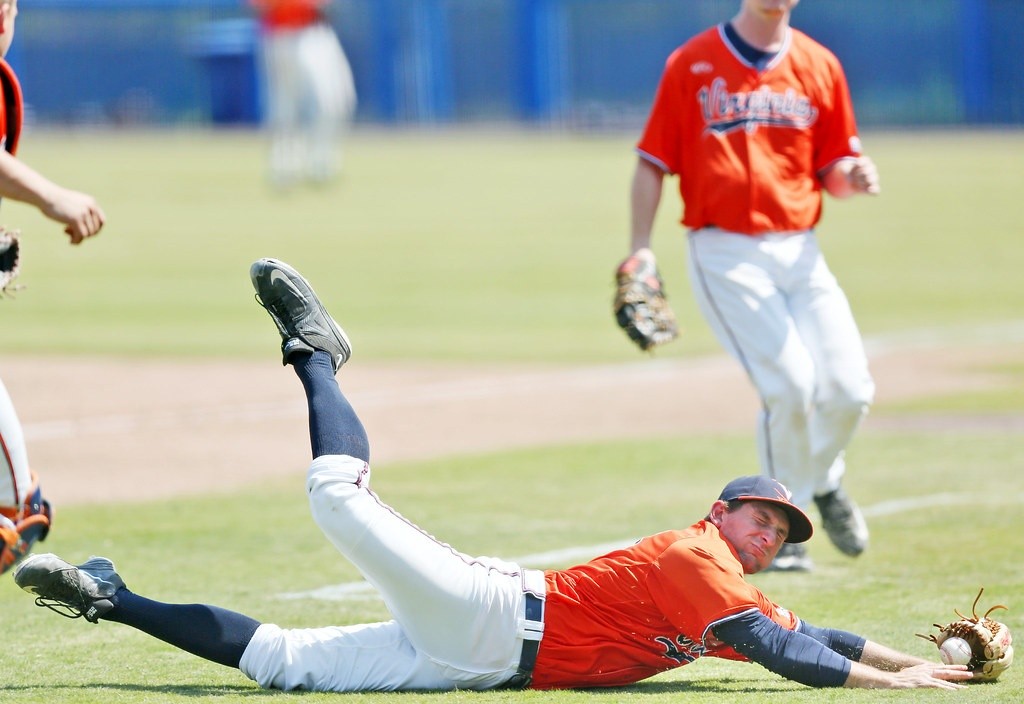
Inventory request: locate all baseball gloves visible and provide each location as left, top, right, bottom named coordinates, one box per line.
left=615, top=255, right=677, bottom=349
left=932, top=618, right=1012, bottom=683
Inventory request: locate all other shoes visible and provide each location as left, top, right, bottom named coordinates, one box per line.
left=767, top=541, right=812, bottom=572
left=813, top=484, right=868, bottom=557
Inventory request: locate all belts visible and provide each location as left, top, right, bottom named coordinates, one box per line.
left=497, top=594, right=542, bottom=690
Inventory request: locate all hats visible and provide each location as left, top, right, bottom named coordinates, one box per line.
left=718, top=475, right=812, bottom=544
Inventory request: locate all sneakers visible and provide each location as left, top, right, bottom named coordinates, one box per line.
left=250, top=257, right=351, bottom=376
left=14, top=553, right=124, bottom=625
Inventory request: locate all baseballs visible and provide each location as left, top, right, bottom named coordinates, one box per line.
left=938, top=637, right=972, bottom=668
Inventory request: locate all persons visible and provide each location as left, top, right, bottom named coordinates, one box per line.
left=614, top=1, right=881, bottom=570
left=248, top=0, right=360, bottom=185
left=4, top=260, right=978, bottom=692
left=0, top=0, right=108, bottom=575
